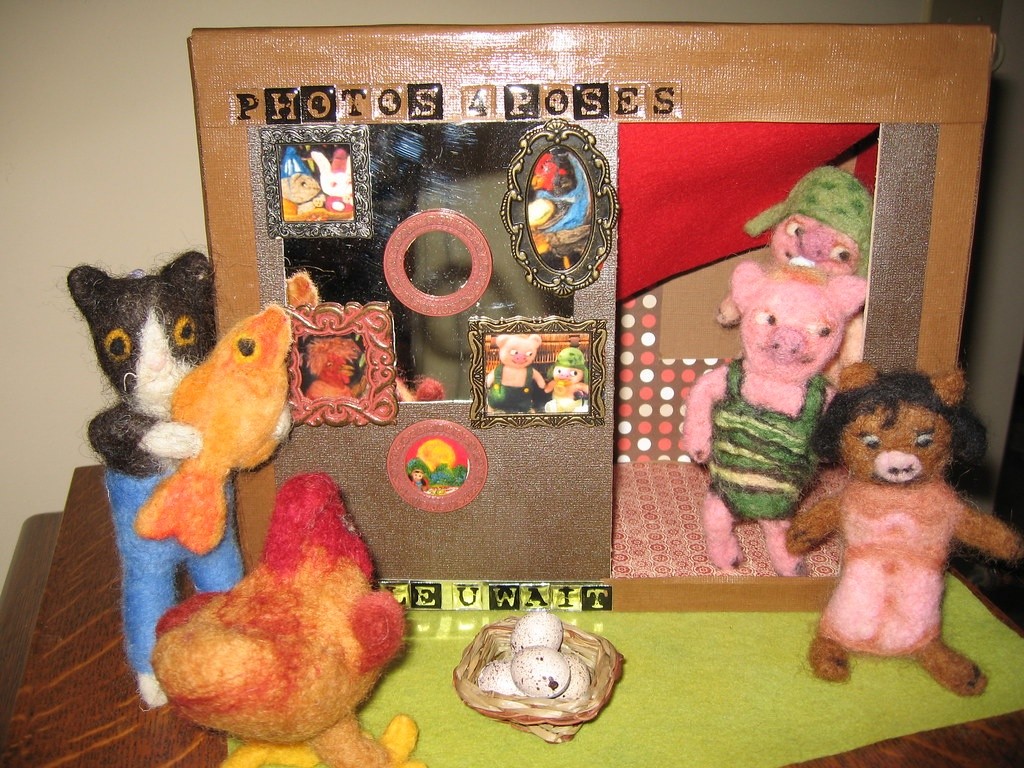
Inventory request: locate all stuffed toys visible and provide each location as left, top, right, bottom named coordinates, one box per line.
left=311, top=150, right=353, bottom=220
left=683, top=259, right=871, bottom=575
left=746, top=167, right=875, bottom=272
left=279, top=146, right=323, bottom=220
left=485, top=334, right=546, bottom=415
left=785, top=366, right=1023, bottom=696
left=152, top=473, right=417, bottom=766
left=65, top=253, right=295, bottom=709
left=544, top=348, right=589, bottom=413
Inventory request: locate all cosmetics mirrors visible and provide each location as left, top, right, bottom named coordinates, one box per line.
left=262, top=125, right=603, bottom=425
